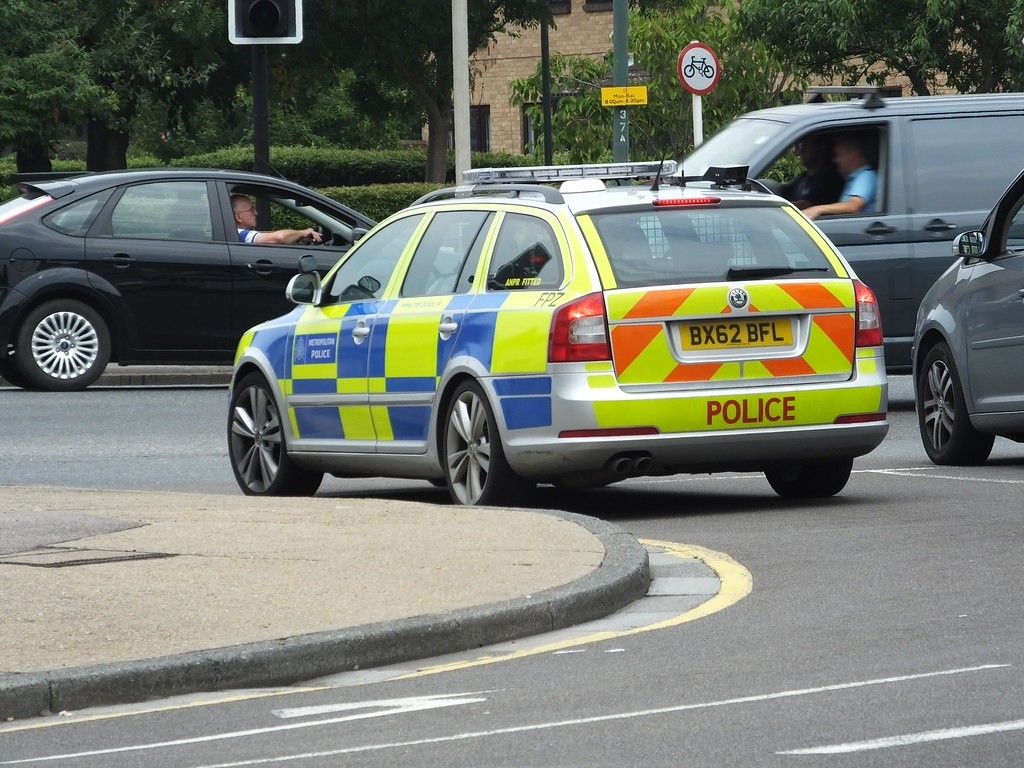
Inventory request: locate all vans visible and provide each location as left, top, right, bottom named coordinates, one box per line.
left=660, top=80, right=1024, bottom=374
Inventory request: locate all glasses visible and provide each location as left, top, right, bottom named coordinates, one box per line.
left=238, top=206, right=254, bottom=215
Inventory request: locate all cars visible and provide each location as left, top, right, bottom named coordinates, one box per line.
left=909, top=168, right=1024, bottom=468
left=225, top=158, right=891, bottom=508
left=0, top=164, right=379, bottom=393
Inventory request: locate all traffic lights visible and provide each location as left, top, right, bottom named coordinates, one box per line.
left=227, top=0, right=305, bottom=45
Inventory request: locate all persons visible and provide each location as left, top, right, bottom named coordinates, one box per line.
left=230, top=194, right=324, bottom=244
left=783, top=135, right=878, bottom=220
